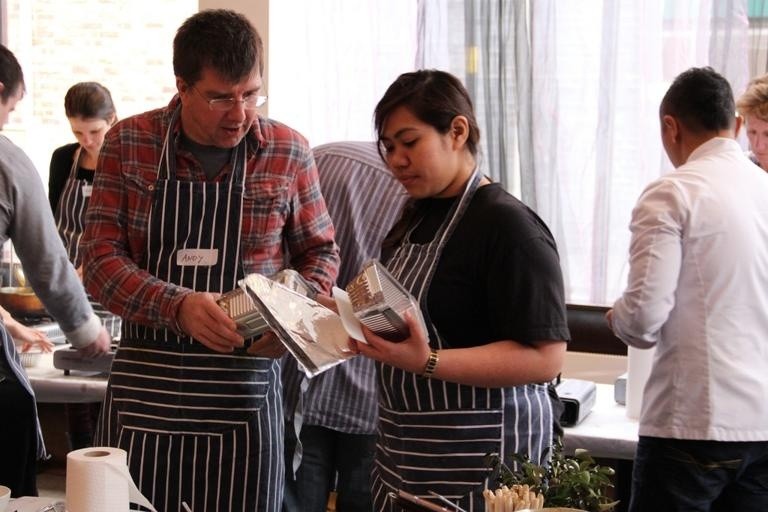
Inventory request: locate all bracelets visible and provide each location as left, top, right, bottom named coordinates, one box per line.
left=417, top=347, right=438, bottom=382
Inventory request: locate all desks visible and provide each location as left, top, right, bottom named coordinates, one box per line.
left=11, top=328, right=639, bottom=509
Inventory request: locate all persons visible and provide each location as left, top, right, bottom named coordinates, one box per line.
left=347, top=71, right=572, bottom=512
left=0, top=45, right=110, bottom=498
left=0, top=305, right=54, bottom=355
left=79, top=10, right=341, bottom=512
left=605, top=67, right=768, bottom=512
left=48, top=82, right=119, bottom=319
left=736, top=75, right=768, bottom=172
left=284, top=141, right=411, bottom=512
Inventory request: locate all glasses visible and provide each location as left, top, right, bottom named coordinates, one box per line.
left=191, top=80, right=266, bottom=111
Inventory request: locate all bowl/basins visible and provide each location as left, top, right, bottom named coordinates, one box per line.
left=0, top=485, right=12, bottom=511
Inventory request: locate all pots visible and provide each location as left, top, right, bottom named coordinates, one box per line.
left=0, top=284, right=48, bottom=315
left=95, top=315, right=120, bottom=341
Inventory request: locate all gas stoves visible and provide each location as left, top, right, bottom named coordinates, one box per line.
left=52, top=338, right=121, bottom=373
